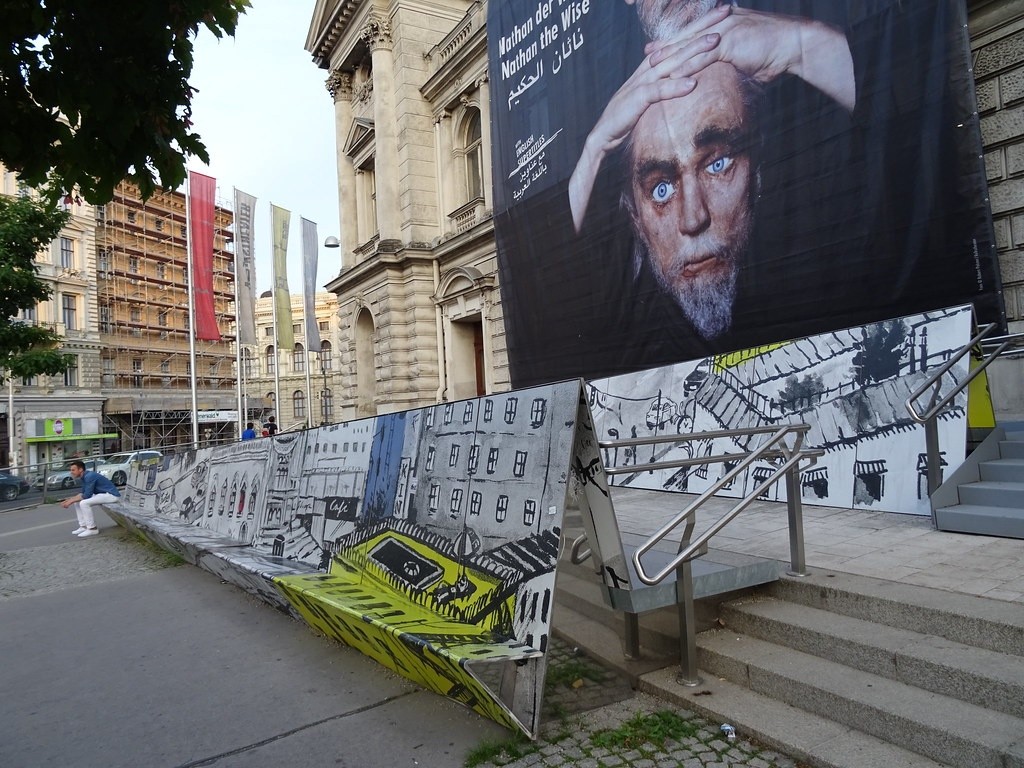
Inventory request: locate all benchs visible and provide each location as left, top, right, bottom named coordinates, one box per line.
left=99, top=374, right=778, bottom=743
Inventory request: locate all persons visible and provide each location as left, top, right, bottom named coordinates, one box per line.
left=61, top=461, right=121, bottom=537
left=262, top=416, right=278, bottom=437
left=242, top=422, right=255, bottom=441
left=547, top=0, right=854, bottom=365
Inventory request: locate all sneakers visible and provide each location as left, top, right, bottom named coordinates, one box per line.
left=78, top=528, right=100, bottom=537
left=72, top=527, right=86, bottom=535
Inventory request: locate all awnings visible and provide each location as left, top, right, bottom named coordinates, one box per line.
left=25, top=433, right=118, bottom=442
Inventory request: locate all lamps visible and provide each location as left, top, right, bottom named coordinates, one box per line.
left=324, top=235, right=341, bottom=248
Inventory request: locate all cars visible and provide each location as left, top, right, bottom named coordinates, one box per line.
left=0, top=471, right=31, bottom=502
left=32, top=458, right=112, bottom=491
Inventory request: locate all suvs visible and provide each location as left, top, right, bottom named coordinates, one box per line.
left=86, top=450, right=165, bottom=486
